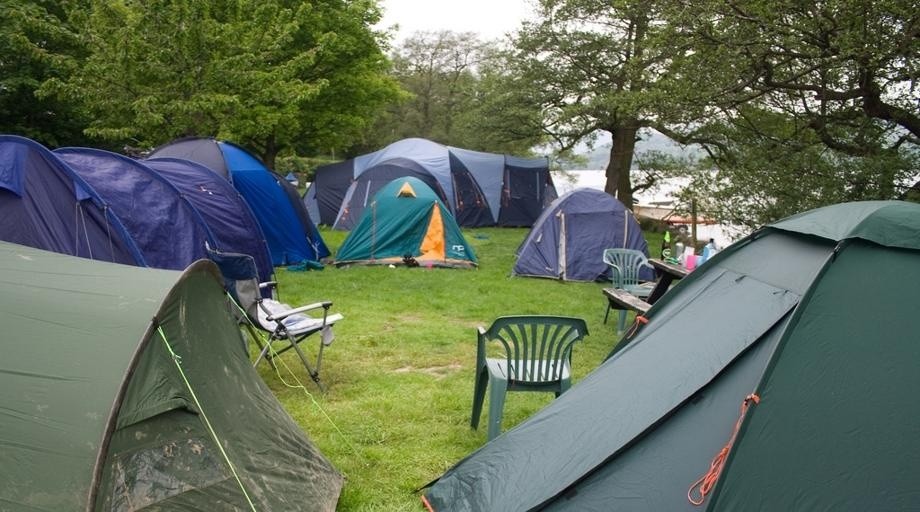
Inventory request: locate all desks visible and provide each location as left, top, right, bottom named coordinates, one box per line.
left=632, top=258, right=689, bottom=328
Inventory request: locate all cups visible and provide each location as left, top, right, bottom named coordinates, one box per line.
left=686, top=255, right=703, bottom=270
left=675, top=242, right=684, bottom=259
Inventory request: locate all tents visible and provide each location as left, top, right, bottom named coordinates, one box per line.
left=0, top=240, right=346, bottom=512
left=0, top=134, right=344, bottom=303
left=421, top=199, right=920, bottom=512
left=331, top=176, right=479, bottom=268
left=302, top=137, right=558, bottom=229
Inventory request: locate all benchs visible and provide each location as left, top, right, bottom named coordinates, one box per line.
left=602, top=286, right=657, bottom=328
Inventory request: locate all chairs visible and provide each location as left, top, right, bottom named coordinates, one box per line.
left=602, top=246, right=657, bottom=337
left=202, top=240, right=346, bottom=393
left=470, top=312, right=590, bottom=446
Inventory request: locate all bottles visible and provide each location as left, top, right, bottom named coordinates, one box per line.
left=703, top=238, right=716, bottom=263
left=662, top=230, right=672, bottom=262
